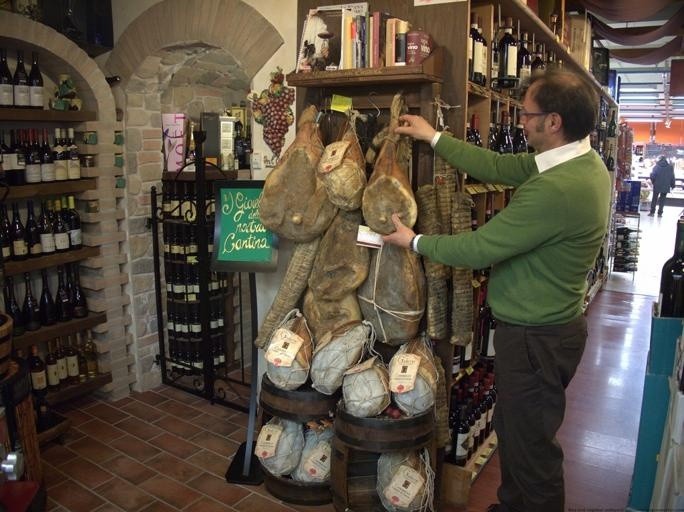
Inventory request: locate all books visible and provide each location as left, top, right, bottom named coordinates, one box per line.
left=295, top=2, right=433, bottom=71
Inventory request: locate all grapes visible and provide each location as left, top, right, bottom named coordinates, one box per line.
left=252, top=85, right=295, bottom=159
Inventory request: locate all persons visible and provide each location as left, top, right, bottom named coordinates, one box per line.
left=648, top=154, right=676, bottom=218
left=383, top=70, right=611, bottom=512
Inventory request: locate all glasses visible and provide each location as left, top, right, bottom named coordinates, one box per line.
left=519, top=107, right=545, bottom=119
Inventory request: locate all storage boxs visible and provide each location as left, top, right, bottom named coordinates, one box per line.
left=648, top=303, right=684, bottom=374
left=637, top=353, right=669, bottom=451
left=625, top=449, right=656, bottom=512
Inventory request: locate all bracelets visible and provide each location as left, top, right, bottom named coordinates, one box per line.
left=408, top=236, right=415, bottom=255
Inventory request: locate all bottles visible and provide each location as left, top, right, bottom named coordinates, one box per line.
left=53, top=128, right=68, bottom=182
left=493, top=30, right=501, bottom=93
left=68, top=195, right=82, bottom=250
left=60, top=129, right=67, bottom=147
left=547, top=49, right=554, bottom=64
left=444, top=367, right=496, bottom=466
left=68, top=128, right=81, bottom=181
left=40, top=268, right=57, bottom=326
left=1, top=202, right=11, bottom=237
left=452, top=345, right=460, bottom=377
left=472, top=114, right=483, bottom=184
left=66, top=263, right=73, bottom=290
left=478, top=16, right=487, bottom=86
left=595, top=131, right=614, bottom=170
left=55, top=265, right=72, bottom=322
left=608, top=110, right=617, bottom=137
left=532, top=44, right=545, bottom=76
left=460, top=326, right=474, bottom=368
left=16, top=349, right=25, bottom=360
left=481, top=287, right=490, bottom=307
left=162, top=181, right=228, bottom=377
left=68, top=336, right=80, bottom=385
left=12, top=50, right=29, bottom=109
left=557, top=59, right=563, bottom=69
left=29, top=52, right=43, bottom=110
left=72, top=262, right=88, bottom=318
left=613, top=227, right=641, bottom=272
left=475, top=292, right=484, bottom=351
left=480, top=308, right=497, bottom=357
left=484, top=200, right=491, bottom=276
left=30, top=345, right=48, bottom=397
left=6, top=276, right=25, bottom=337
left=471, top=12, right=484, bottom=84
left=498, top=17, right=518, bottom=88
left=76, top=333, right=89, bottom=383
left=41, top=128, right=55, bottom=184
left=53, top=200, right=70, bottom=253
left=0, top=130, right=11, bottom=187
left=595, top=247, right=605, bottom=274
left=55, top=338, right=69, bottom=389
left=495, top=111, right=513, bottom=153
left=234, top=121, right=246, bottom=171
left=61, top=196, right=68, bottom=222
left=23, top=272, right=42, bottom=331
left=11, top=128, right=42, bottom=186
left=468, top=32, right=474, bottom=81
left=514, top=124, right=529, bottom=154
left=45, top=341, right=60, bottom=392
left=38, top=199, right=56, bottom=256
left=517, top=33, right=532, bottom=96
left=600, top=96, right=611, bottom=129
left=11, top=201, right=29, bottom=262
left=59, top=336, right=65, bottom=353
left=47, top=200, right=55, bottom=223
left=487, top=123, right=499, bottom=150
left=0, top=209, right=11, bottom=263
left=658, top=216, right=684, bottom=318
left=464, top=122, right=475, bottom=185
left=471, top=210, right=480, bottom=279
left=26, top=200, right=42, bottom=258
left=0, top=48, right=14, bottom=108
left=85, top=329, right=99, bottom=378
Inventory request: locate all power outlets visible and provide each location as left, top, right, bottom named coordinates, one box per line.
left=1, top=106, right=99, bottom=406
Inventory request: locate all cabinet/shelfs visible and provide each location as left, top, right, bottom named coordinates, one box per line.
left=298, top=1, right=614, bottom=510
left=335, top=400, right=437, bottom=512
left=287, top=45, right=448, bottom=362
left=162, top=171, right=238, bottom=372
left=612, top=211, right=643, bottom=280
left=258, top=371, right=340, bottom=505
